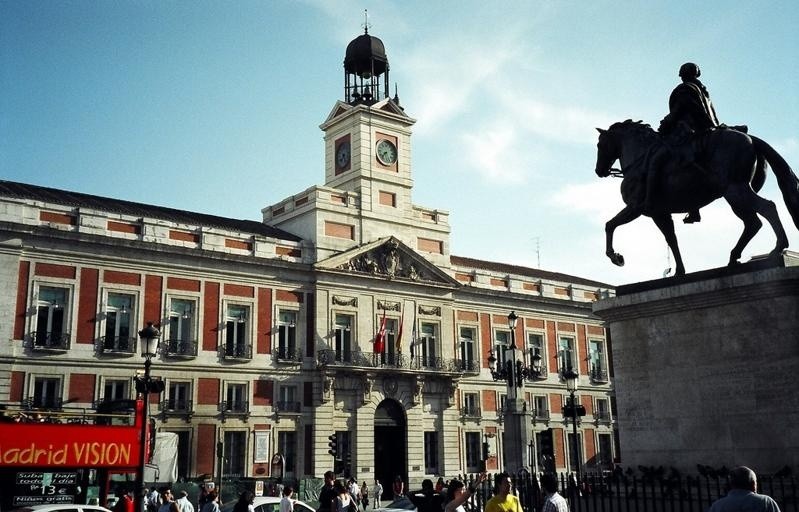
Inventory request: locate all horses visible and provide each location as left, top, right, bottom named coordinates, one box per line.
left=595, top=119, right=799, bottom=276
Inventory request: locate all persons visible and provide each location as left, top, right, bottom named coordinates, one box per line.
left=360, top=480, right=370, bottom=511
left=540, top=472, right=569, bottom=512
left=660, top=61, right=718, bottom=225
left=372, top=480, right=383, bottom=510
left=316, top=471, right=360, bottom=511
left=435, top=477, right=449, bottom=492
left=543, top=454, right=555, bottom=472
left=540, top=453, right=547, bottom=470
left=137, top=495, right=138, bottom=496
left=392, top=474, right=405, bottom=502
left=279, top=486, right=295, bottom=511
left=444, top=472, right=488, bottom=511
left=485, top=473, right=524, bottom=512
left=709, top=465, right=780, bottom=512
left=143, top=480, right=223, bottom=512
left=111, top=484, right=135, bottom=512
left=263, top=477, right=284, bottom=497
left=232, top=490, right=256, bottom=512
left=406, top=479, right=447, bottom=511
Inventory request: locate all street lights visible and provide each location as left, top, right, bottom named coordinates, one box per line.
left=561, top=359, right=583, bottom=482
left=487, top=310, right=545, bottom=400
left=135, top=320, right=160, bottom=510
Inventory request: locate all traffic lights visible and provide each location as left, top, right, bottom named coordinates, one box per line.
left=485, top=441, right=491, bottom=460
left=327, top=435, right=338, bottom=456
left=345, top=451, right=352, bottom=470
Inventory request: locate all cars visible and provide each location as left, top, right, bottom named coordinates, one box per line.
left=21, top=503, right=113, bottom=511
left=220, top=497, right=318, bottom=512
left=363, top=493, right=480, bottom=510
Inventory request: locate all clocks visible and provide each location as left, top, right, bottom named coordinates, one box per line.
left=335, top=142, right=351, bottom=169
left=375, top=138, right=399, bottom=166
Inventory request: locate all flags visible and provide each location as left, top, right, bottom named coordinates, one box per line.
left=396, top=305, right=405, bottom=353
left=373, top=308, right=386, bottom=354
left=409, top=310, right=416, bottom=369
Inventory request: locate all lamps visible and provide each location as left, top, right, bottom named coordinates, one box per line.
left=120, top=305, right=127, bottom=314
left=390, top=328, right=395, bottom=335
left=289, top=320, right=296, bottom=329
left=237, top=315, right=245, bottom=323
left=345, top=324, right=351, bottom=332
left=430, top=332, right=436, bottom=340
left=51, top=299, right=59, bottom=309
left=181, top=310, right=189, bottom=320
